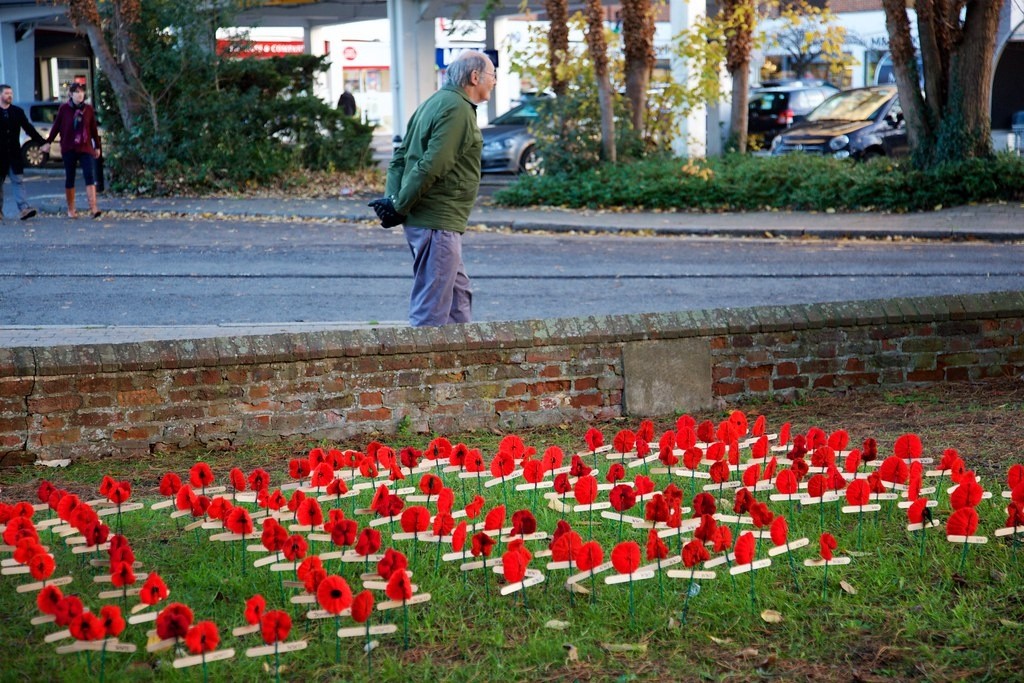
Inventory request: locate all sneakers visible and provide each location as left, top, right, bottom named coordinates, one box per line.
left=19, top=208, right=37, bottom=220
left=0, top=212, right=4, bottom=220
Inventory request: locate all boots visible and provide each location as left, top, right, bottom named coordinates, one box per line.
left=86, top=184, right=102, bottom=219
left=65, top=187, right=78, bottom=218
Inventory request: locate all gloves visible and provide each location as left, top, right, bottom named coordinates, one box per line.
left=366, top=198, right=407, bottom=228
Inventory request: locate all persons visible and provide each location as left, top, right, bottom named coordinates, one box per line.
left=39, top=83, right=102, bottom=220
left=368, top=52, right=498, bottom=326
left=338, top=84, right=356, bottom=119
left=0, top=84, right=52, bottom=220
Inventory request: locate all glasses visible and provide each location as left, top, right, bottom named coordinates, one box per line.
left=480, top=69, right=497, bottom=81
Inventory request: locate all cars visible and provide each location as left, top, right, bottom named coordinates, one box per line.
left=770, top=86, right=913, bottom=165
left=14, top=101, right=126, bottom=168
left=479, top=96, right=631, bottom=182
left=746, top=85, right=846, bottom=149
